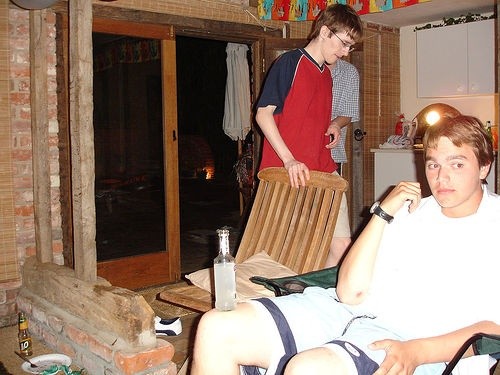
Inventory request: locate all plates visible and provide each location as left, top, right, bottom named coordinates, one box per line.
left=22, top=353, right=72, bottom=375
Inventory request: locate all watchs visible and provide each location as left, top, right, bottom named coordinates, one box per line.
left=370, top=201, right=394, bottom=224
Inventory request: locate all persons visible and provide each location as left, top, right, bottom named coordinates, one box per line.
left=188, top=113, right=500, bottom=374
left=253, top=4, right=365, bottom=269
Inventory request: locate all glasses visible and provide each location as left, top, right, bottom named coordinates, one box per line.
left=329, top=28, right=355, bottom=52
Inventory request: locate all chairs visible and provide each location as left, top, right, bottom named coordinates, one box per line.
left=157, top=166, right=350, bottom=375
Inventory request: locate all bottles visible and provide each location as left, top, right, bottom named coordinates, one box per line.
left=394, top=115, right=406, bottom=136
left=486, top=121, right=492, bottom=143
left=212, top=228, right=238, bottom=312
left=17, top=312, right=34, bottom=356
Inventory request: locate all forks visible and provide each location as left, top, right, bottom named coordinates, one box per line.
left=14, top=351, right=39, bottom=369
